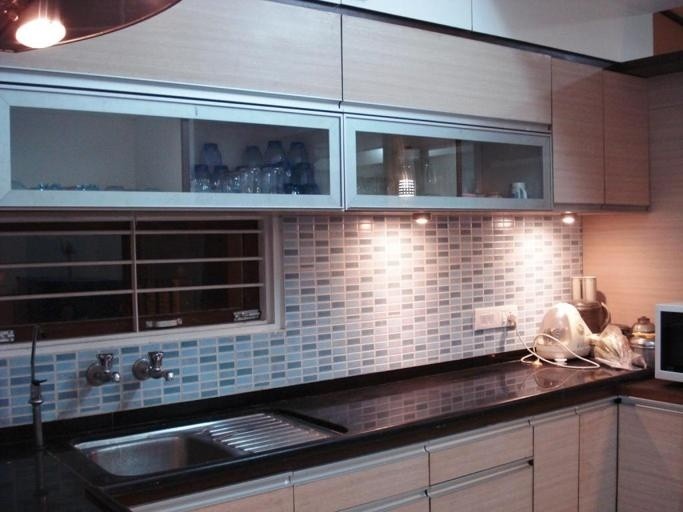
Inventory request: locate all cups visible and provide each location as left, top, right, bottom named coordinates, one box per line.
left=190, top=139, right=317, bottom=195
left=508, top=182, right=529, bottom=200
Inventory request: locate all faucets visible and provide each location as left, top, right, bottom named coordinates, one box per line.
left=134, top=352, right=174, bottom=384
left=86, top=353, right=120, bottom=387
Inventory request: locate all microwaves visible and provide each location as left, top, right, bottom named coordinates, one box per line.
left=655, top=304, right=683, bottom=382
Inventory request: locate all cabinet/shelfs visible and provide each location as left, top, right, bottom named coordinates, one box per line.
left=552, top=57, right=652, bottom=212
left=0, top=0, right=553, bottom=215
left=532, top=404, right=682, bottom=512
left=293, top=419, right=532, bottom=512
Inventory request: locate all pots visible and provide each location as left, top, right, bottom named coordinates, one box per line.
left=627, top=338, right=654, bottom=367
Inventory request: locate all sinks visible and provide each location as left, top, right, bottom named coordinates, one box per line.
left=58, top=430, right=242, bottom=491
